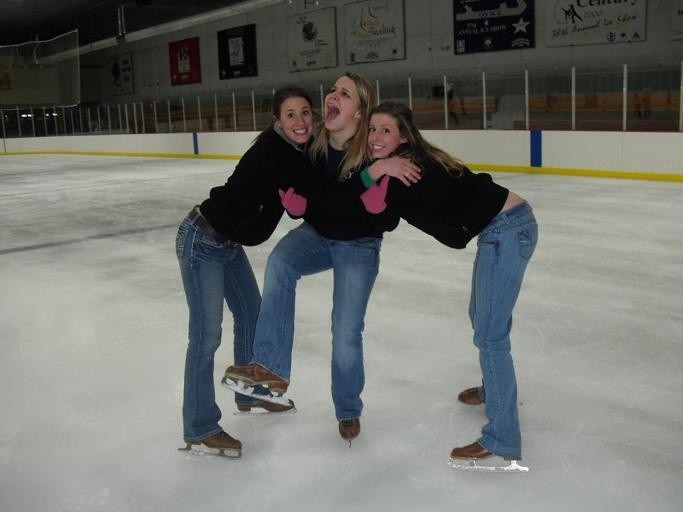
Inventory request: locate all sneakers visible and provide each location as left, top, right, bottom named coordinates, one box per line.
left=339, top=417, right=360, bottom=440
left=226, top=363, right=288, bottom=392
left=186, top=431, right=242, bottom=451
left=235, top=397, right=294, bottom=413
left=451, top=441, right=520, bottom=460
left=459, top=387, right=482, bottom=405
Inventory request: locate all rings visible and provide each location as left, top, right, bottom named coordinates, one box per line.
left=404, top=172, right=410, bottom=178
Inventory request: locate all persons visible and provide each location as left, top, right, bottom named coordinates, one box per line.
left=303, top=102, right=538, bottom=461
left=224, top=71, right=400, bottom=441
left=174, top=87, right=422, bottom=451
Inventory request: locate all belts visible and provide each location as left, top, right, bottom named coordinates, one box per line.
left=191, top=204, right=242, bottom=249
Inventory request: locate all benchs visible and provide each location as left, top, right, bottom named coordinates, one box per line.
left=377, top=89, right=680, bottom=133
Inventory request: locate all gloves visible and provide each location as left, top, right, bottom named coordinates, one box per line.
left=279, top=187, right=307, bottom=216
left=360, top=175, right=390, bottom=214
